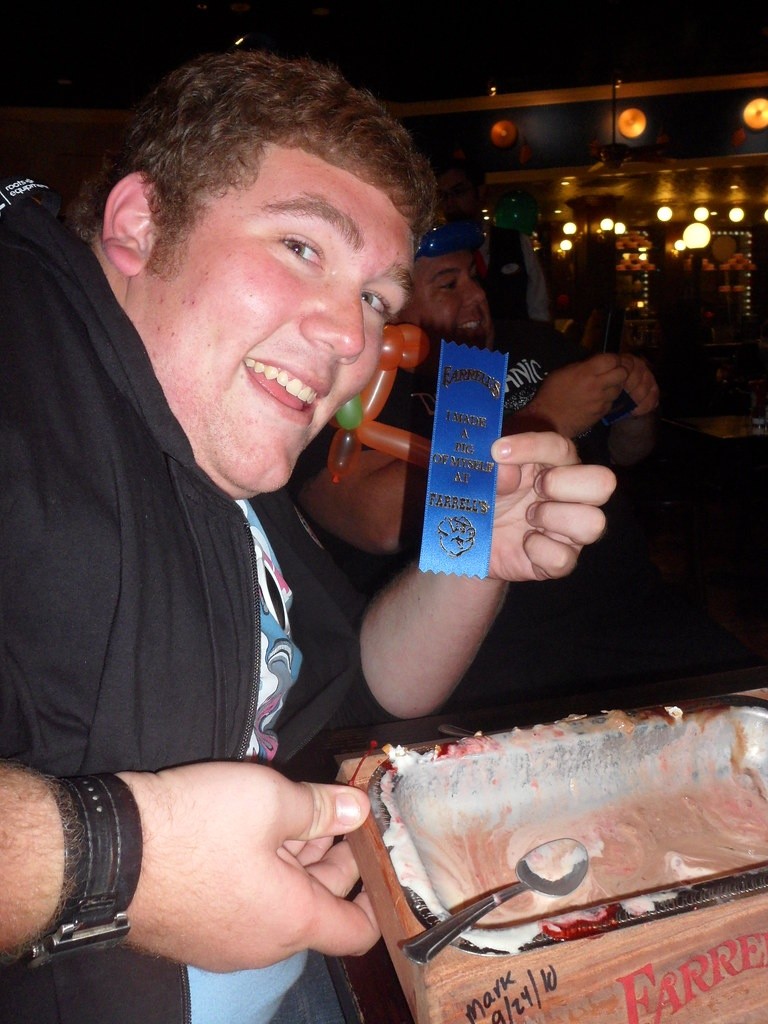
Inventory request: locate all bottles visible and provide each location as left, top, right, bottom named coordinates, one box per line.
left=704, top=312, right=715, bottom=344
left=630, top=323, right=640, bottom=345
left=647, top=321, right=656, bottom=344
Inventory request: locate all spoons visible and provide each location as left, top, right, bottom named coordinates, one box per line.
left=403, top=838, right=588, bottom=963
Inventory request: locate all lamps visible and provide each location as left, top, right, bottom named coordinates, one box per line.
left=595, top=82, right=631, bottom=169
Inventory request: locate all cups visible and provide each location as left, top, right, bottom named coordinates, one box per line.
left=751, top=382, right=768, bottom=428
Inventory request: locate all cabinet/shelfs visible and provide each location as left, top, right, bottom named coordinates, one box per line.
left=581, top=222, right=768, bottom=366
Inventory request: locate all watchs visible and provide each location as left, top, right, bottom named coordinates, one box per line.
left=6, top=773, right=143, bottom=978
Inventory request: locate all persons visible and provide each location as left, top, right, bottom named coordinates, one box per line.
left=0, top=52, right=662, bottom=1023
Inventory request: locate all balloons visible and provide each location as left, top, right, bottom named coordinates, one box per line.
left=326, top=324, right=432, bottom=485
